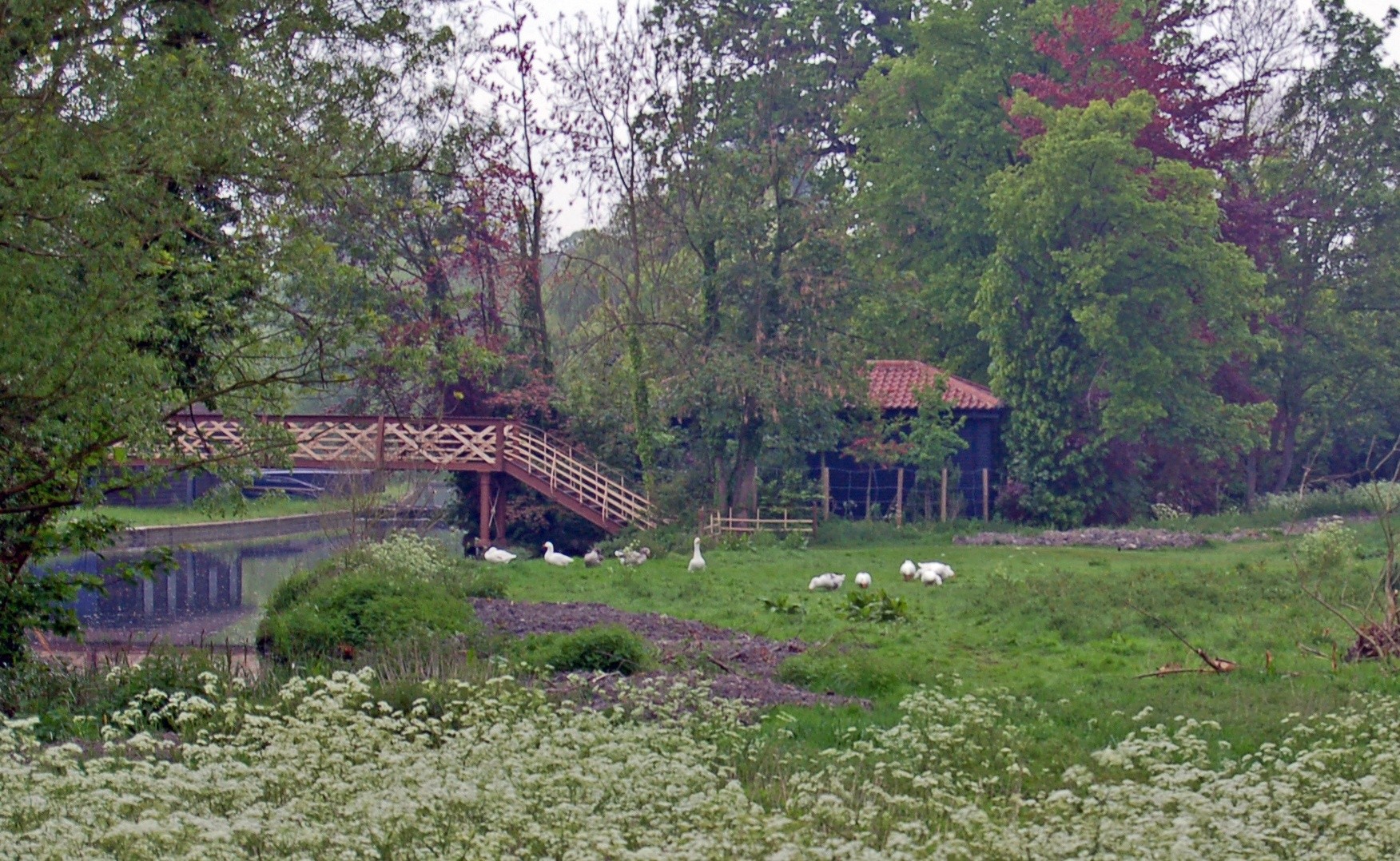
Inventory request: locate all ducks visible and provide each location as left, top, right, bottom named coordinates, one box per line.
left=484, top=545, right=518, bottom=564
left=543, top=541, right=574, bottom=567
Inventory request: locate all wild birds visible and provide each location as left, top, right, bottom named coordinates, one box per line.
left=584, top=546, right=651, bottom=566
left=687, top=537, right=706, bottom=574
left=808, top=559, right=956, bottom=595
